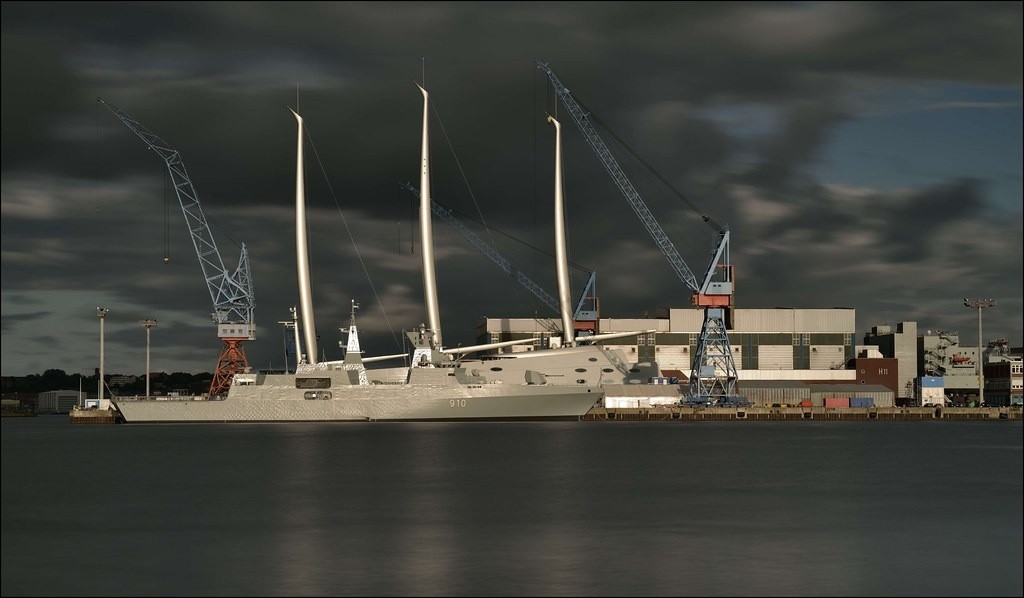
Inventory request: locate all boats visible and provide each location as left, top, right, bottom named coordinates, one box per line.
left=65, top=78, right=608, bottom=421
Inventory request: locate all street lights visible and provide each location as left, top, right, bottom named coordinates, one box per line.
left=94, top=304, right=109, bottom=399
left=137, top=318, right=157, bottom=399
left=963, top=296, right=997, bottom=407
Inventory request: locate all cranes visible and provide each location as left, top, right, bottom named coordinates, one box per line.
left=394, top=177, right=604, bottom=340
left=537, top=55, right=754, bottom=412
left=95, top=92, right=255, bottom=399
